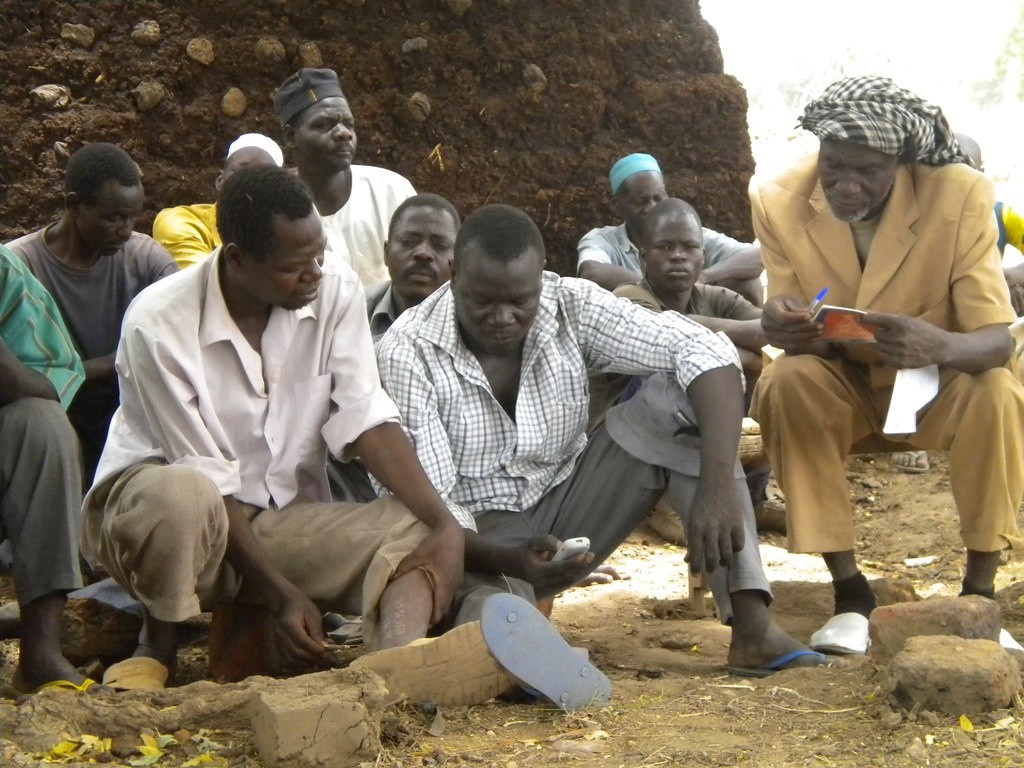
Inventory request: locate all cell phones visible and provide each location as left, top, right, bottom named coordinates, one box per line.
left=551, top=537, right=590, bottom=563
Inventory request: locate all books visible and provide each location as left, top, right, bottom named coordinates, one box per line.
left=813, top=305, right=875, bottom=343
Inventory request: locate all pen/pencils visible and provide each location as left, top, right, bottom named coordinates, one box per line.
left=806, top=288, right=829, bottom=312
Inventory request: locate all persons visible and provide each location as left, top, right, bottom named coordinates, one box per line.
left=0, top=67, right=836, bottom=713
left=950, top=132, right=1024, bottom=262
left=747, top=76, right=1024, bottom=657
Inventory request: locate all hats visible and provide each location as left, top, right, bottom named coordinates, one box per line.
left=608, top=152, right=662, bottom=194
left=226, top=133, right=284, bottom=168
left=794, top=76, right=974, bottom=168
left=273, top=68, right=346, bottom=128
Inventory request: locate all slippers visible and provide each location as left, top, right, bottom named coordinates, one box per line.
left=725, top=650, right=849, bottom=678
left=349, top=620, right=517, bottom=706
left=4, top=678, right=116, bottom=701
left=102, top=656, right=178, bottom=691
left=480, top=592, right=613, bottom=712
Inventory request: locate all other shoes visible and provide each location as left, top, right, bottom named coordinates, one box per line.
left=999, top=628, right=1024, bottom=651
left=810, top=611, right=871, bottom=655
